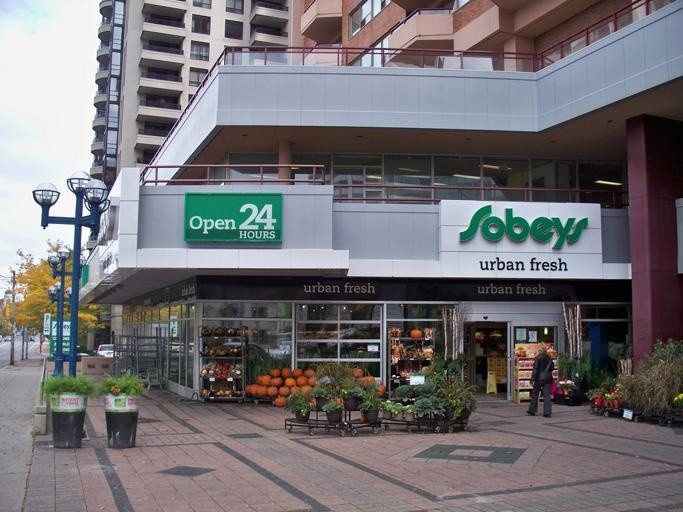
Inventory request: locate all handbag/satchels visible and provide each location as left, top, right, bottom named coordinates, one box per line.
left=538, top=369, right=552, bottom=381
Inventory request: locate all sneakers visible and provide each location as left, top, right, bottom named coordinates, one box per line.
left=542, top=412, right=552, bottom=418
left=526, top=409, right=537, bottom=416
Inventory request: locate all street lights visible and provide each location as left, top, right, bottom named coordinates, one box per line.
left=31, top=170, right=113, bottom=384
left=48, top=282, right=72, bottom=379
left=48, top=244, right=89, bottom=378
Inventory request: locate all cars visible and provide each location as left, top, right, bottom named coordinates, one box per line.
left=0, top=328, right=39, bottom=341
left=96, top=342, right=119, bottom=359
left=170, top=330, right=291, bottom=363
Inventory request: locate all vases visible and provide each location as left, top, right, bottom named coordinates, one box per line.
left=612, top=400, right=622, bottom=409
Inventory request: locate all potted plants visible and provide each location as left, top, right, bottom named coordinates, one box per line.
left=557, top=382, right=576, bottom=395
left=97, top=368, right=148, bottom=448
left=282, top=351, right=480, bottom=421
left=584, top=376, right=616, bottom=409
left=42, top=374, right=97, bottom=448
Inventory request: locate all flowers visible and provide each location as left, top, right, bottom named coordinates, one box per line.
left=604, top=391, right=624, bottom=400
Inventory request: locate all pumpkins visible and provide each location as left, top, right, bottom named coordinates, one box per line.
left=245, top=368, right=387, bottom=407
left=410, top=329, right=424, bottom=338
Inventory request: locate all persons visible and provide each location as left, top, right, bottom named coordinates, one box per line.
left=524, top=341, right=554, bottom=418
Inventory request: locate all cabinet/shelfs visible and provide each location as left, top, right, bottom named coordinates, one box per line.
left=488, top=357, right=507, bottom=393
left=514, top=356, right=559, bottom=404
